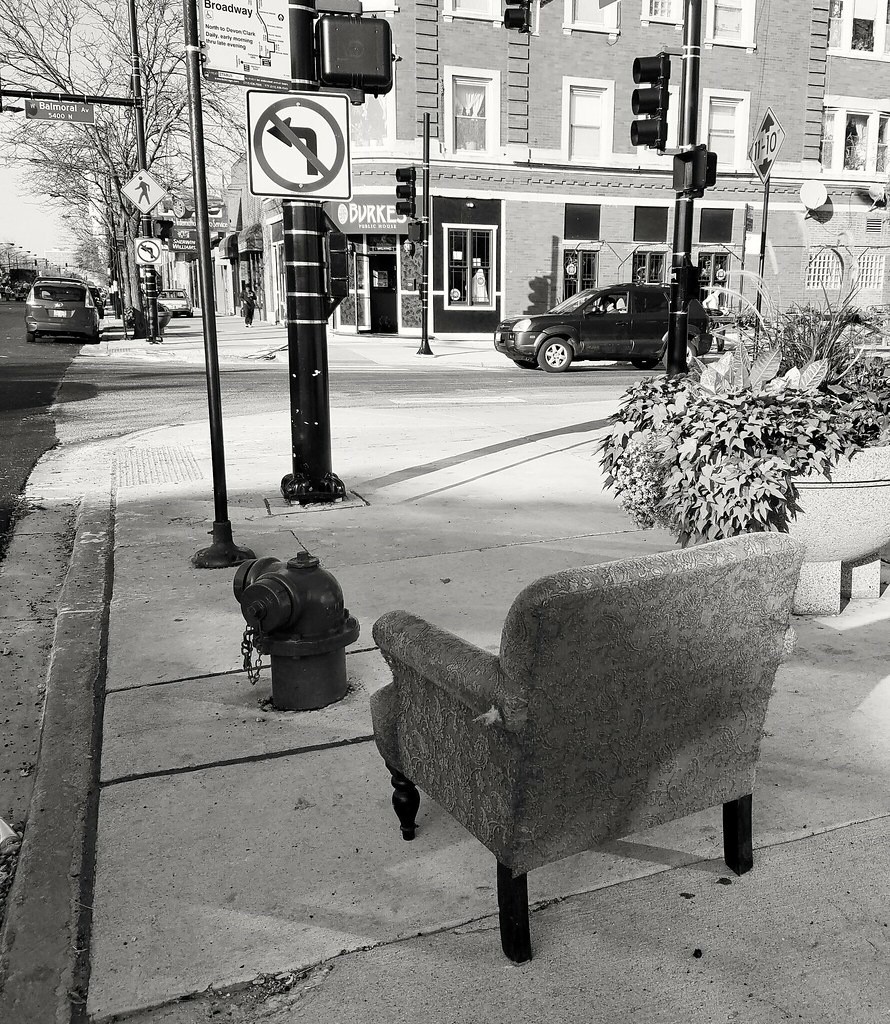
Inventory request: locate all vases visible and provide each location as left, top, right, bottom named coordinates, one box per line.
left=465, top=141, right=477, bottom=150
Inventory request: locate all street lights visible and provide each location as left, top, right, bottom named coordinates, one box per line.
left=16, top=253, right=37, bottom=270
left=7, top=250, right=32, bottom=271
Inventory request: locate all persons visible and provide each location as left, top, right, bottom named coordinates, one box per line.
left=846, top=116, right=857, bottom=161
left=239, top=284, right=257, bottom=327
left=0, top=281, right=29, bottom=298
left=599, top=297, right=615, bottom=314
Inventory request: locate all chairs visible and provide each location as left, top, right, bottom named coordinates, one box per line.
left=161, top=293, right=166, bottom=298
left=370, top=532, right=805, bottom=963
left=616, top=298, right=626, bottom=309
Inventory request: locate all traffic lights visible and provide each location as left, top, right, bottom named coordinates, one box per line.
left=630, top=51, right=671, bottom=149
left=503, top=0, right=534, bottom=35
left=151, top=219, right=175, bottom=239
left=396, top=167, right=418, bottom=216
left=672, top=145, right=720, bottom=193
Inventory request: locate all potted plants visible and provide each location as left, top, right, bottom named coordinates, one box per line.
left=593, top=233, right=890, bottom=614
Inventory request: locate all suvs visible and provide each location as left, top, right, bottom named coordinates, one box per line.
left=494, top=283, right=711, bottom=374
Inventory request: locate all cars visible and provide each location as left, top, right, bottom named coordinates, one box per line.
left=24, top=280, right=101, bottom=344
left=154, top=288, right=195, bottom=317
left=30, top=276, right=108, bottom=320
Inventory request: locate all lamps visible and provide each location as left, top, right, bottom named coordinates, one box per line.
left=348, top=241, right=352, bottom=254
left=403, top=239, right=412, bottom=257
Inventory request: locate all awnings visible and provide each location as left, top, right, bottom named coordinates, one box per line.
left=218, top=234, right=238, bottom=258
left=238, top=223, right=263, bottom=253
